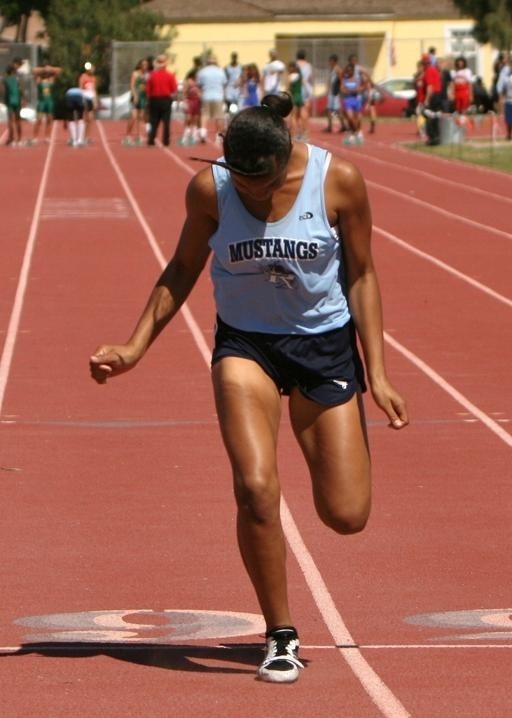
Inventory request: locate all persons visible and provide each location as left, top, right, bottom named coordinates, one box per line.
left=121, top=48, right=380, bottom=147
left=87, top=90, right=409, bottom=685
left=409, top=46, right=512, bottom=146
left=0, top=56, right=99, bottom=150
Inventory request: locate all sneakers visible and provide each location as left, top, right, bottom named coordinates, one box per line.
left=259, top=626, right=304, bottom=681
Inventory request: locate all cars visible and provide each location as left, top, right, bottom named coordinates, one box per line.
left=308, top=82, right=410, bottom=118
left=97, top=84, right=239, bottom=123
left=373, top=76, right=417, bottom=100
left=0, top=101, right=36, bottom=124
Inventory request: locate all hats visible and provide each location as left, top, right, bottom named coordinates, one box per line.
left=154, top=55, right=169, bottom=66
left=423, top=56, right=430, bottom=63
